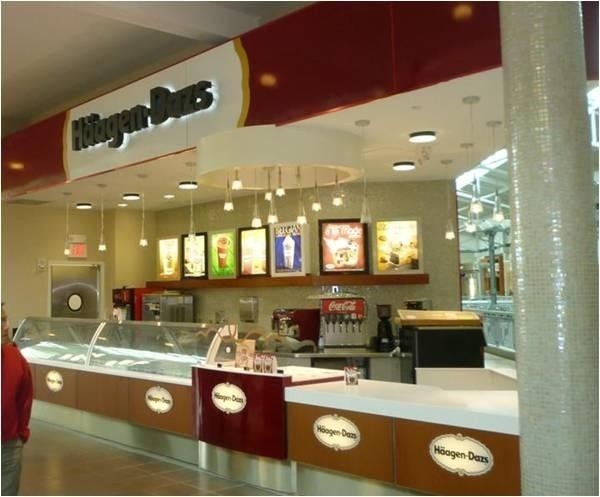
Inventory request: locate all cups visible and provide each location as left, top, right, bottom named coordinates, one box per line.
left=283, top=241, right=295, bottom=268
left=216, top=236, right=232, bottom=268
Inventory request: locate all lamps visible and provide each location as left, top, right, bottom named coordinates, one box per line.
left=63, top=158, right=343, bottom=255
left=391, top=95, right=504, bottom=242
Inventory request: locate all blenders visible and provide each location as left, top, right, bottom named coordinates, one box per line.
left=375, top=304, right=396, bottom=352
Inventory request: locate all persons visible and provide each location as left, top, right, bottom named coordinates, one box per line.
left=0, top=298, right=34, bottom=495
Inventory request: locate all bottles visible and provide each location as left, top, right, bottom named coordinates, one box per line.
left=252, top=353, right=278, bottom=374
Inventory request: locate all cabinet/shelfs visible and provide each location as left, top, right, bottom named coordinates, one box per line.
left=11, top=315, right=238, bottom=440
